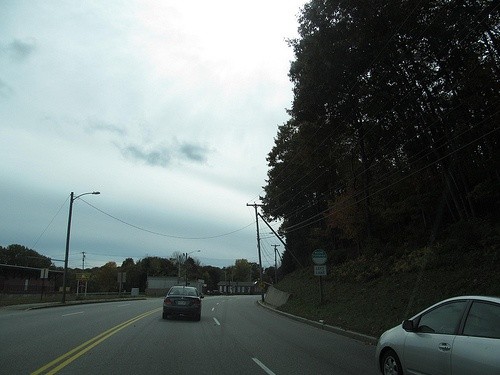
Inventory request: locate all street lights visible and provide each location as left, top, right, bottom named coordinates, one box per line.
left=186, top=249, right=201, bottom=286
left=61, top=191, right=100, bottom=304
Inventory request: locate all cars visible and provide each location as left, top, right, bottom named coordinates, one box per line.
left=163, top=286, right=204, bottom=322
left=375, top=295, right=500, bottom=375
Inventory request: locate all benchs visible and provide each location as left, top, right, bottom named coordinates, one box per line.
left=175, top=291, right=195, bottom=296
